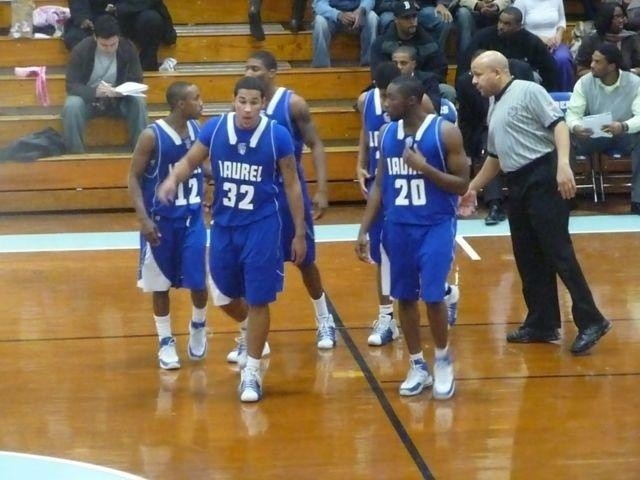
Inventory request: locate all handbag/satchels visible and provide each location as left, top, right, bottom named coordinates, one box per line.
left=14, top=66, right=49, bottom=106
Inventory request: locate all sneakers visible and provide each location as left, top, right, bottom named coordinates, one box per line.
left=316, top=315, right=337, bottom=349
left=432, top=359, right=456, bottom=400
left=228, top=335, right=270, bottom=361
left=188, top=321, right=207, bottom=360
left=443, top=286, right=459, bottom=329
left=367, top=317, right=399, bottom=345
left=236, top=319, right=253, bottom=362
left=158, top=338, right=181, bottom=369
left=399, top=359, right=433, bottom=396
left=238, top=366, right=264, bottom=401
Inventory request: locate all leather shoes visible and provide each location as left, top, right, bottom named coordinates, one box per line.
left=249, top=7, right=264, bottom=41
left=291, top=19, right=304, bottom=31
left=506, top=324, right=562, bottom=342
left=570, top=319, right=612, bottom=354
left=485, top=206, right=505, bottom=224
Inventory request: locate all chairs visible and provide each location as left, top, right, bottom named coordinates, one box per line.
left=546, top=92, right=599, bottom=208
left=598, top=148, right=633, bottom=207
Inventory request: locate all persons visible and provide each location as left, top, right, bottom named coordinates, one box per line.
left=209, top=53, right=339, bottom=363
left=577, top=3, right=640, bottom=79
left=376, top=0, right=452, bottom=53
left=358, top=63, right=459, bottom=362
left=248, top=0, right=305, bottom=40
left=391, top=45, right=441, bottom=112
left=157, top=78, right=308, bottom=402
left=452, top=0, right=518, bottom=52
left=513, top=0, right=576, bottom=92
left=457, top=58, right=545, bottom=225
left=129, top=82, right=213, bottom=370
left=456, top=50, right=612, bottom=354
left=62, top=14, right=148, bottom=154
left=370, top=2, right=447, bottom=84
left=310, top=0, right=380, bottom=69
left=356, top=74, right=469, bottom=398
left=566, top=45, right=640, bottom=213
left=471, top=8, right=556, bottom=87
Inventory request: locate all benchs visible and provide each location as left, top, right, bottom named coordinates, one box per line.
left=0, top=0, right=322, bottom=30
left=0, top=147, right=367, bottom=213
left=0, top=31, right=362, bottom=70
left=0, top=106, right=363, bottom=143
left=0, top=64, right=456, bottom=109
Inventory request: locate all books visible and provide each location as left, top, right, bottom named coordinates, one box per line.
left=102, top=79, right=148, bottom=99
left=583, top=112, right=613, bottom=137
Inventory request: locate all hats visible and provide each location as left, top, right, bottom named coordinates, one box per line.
left=393, top=1, right=417, bottom=17
